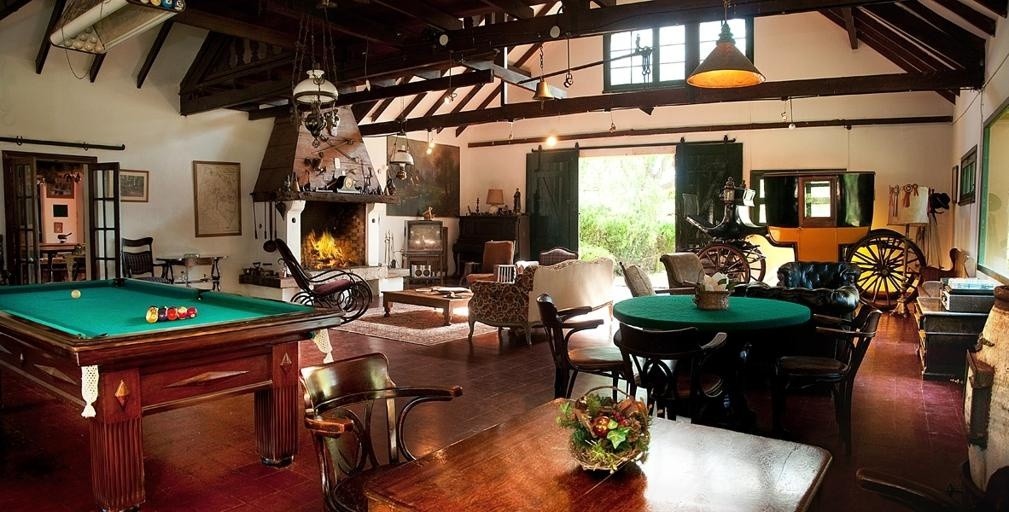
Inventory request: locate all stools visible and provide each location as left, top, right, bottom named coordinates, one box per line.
left=67, top=254, right=85, bottom=281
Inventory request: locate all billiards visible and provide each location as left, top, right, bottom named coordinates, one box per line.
left=72, top=289, right=81, bottom=299
left=146, top=304, right=198, bottom=323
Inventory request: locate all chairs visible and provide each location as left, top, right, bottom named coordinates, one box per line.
left=659, top=252, right=708, bottom=294
left=854, top=466, right=1009, bottom=511
left=774, top=308, right=882, bottom=452
left=537, top=293, right=635, bottom=404
left=297, top=354, right=462, bottom=510
left=121, top=237, right=169, bottom=283
left=538, top=250, right=577, bottom=267
left=614, top=322, right=753, bottom=436
left=264, top=239, right=372, bottom=325
left=917, top=248, right=972, bottom=297
left=465, top=240, right=515, bottom=283
left=619, top=262, right=696, bottom=296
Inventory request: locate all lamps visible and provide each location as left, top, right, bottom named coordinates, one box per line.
left=532, top=51, right=554, bottom=111
left=390, top=128, right=414, bottom=180
left=685, top=0, right=765, bottom=88
left=486, top=189, right=504, bottom=215
left=291, top=0, right=340, bottom=148
left=444, top=54, right=456, bottom=103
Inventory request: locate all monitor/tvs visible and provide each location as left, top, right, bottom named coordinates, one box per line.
left=405, top=220, right=442, bottom=253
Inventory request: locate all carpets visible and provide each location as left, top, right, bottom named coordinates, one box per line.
left=314, top=297, right=510, bottom=348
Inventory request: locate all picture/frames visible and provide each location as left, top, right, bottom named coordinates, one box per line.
left=54, top=222, right=62, bottom=233
left=106, top=169, right=149, bottom=202
left=193, top=160, right=242, bottom=237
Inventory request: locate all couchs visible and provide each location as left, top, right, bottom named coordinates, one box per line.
left=468, top=257, right=615, bottom=348
left=735, top=262, right=860, bottom=330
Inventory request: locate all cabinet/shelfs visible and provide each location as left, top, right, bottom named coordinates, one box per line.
left=403, top=254, right=444, bottom=287
left=914, top=297, right=989, bottom=383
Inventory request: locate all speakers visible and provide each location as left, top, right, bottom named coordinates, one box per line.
left=423, top=265, right=432, bottom=278
left=411, top=265, right=423, bottom=278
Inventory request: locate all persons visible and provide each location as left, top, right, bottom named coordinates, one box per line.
left=514, top=188, right=520, bottom=212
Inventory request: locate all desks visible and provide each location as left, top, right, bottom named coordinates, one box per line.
left=157, top=256, right=228, bottom=292
left=0, top=276, right=344, bottom=512
left=363, top=400, right=833, bottom=510
left=23, top=243, right=85, bottom=283
left=615, top=295, right=810, bottom=428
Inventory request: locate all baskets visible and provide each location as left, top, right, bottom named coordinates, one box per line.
left=695, top=266, right=735, bottom=312
left=569, top=385, right=650, bottom=473
